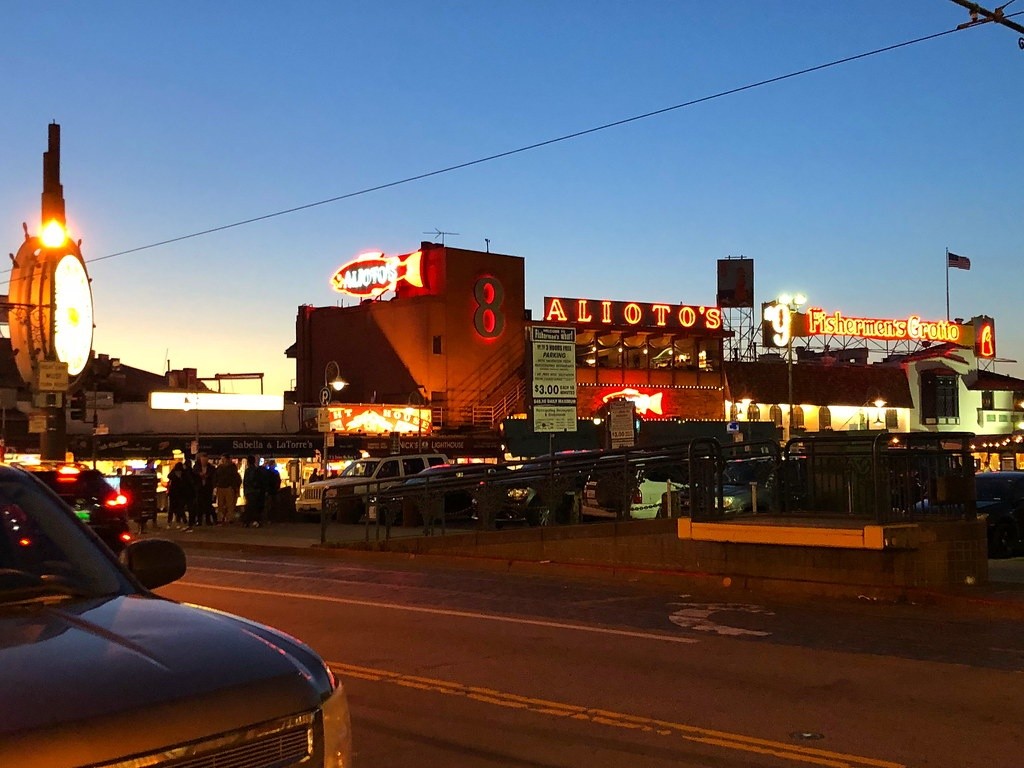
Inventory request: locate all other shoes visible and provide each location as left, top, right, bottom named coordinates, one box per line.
left=166, top=524, right=171, bottom=530
left=206, top=521, right=213, bottom=527
left=185, top=528, right=194, bottom=533
left=216, top=524, right=223, bottom=528
left=176, top=525, right=181, bottom=529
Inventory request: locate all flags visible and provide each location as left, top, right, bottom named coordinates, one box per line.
left=949, top=253, right=971, bottom=270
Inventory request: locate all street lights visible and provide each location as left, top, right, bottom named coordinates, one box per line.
left=321, top=360, right=350, bottom=484
left=34, top=216, right=67, bottom=360
left=776, top=288, right=810, bottom=433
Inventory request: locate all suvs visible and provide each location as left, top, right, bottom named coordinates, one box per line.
left=0, top=459, right=135, bottom=571
left=294, top=452, right=451, bottom=526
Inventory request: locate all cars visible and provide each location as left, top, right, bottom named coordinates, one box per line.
left=898, top=470, right=1024, bottom=559
left=365, top=462, right=513, bottom=527
left=469, top=449, right=808, bottom=526
left=0, top=462, right=356, bottom=767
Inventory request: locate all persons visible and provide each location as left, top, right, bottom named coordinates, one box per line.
left=309, top=468, right=318, bottom=483
left=116, top=452, right=282, bottom=535
left=982, top=461, right=992, bottom=472
